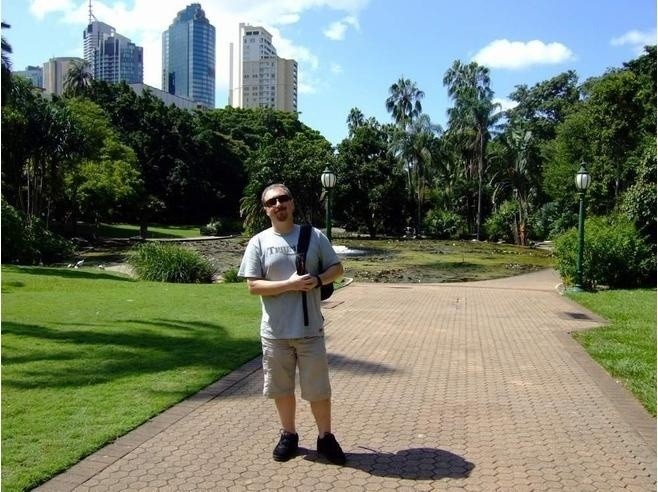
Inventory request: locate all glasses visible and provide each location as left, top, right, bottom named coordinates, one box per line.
left=264, top=194, right=291, bottom=208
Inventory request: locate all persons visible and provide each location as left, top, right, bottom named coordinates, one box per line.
left=242, top=181, right=349, bottom=465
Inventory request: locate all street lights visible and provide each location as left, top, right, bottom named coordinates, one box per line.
left=573, top=159, right=592, bottom=292
left=321, top=160, right=336, bottom=241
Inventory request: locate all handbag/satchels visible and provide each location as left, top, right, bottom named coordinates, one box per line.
left=295, top=225, right=335, bottom=300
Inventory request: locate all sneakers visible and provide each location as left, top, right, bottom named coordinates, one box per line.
left=273, top=432, right=299, bottom=462
left=317, top=432, right=347, bottom=467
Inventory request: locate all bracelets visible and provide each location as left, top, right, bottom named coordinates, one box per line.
left=312, top=274, right=322, bottom=289
